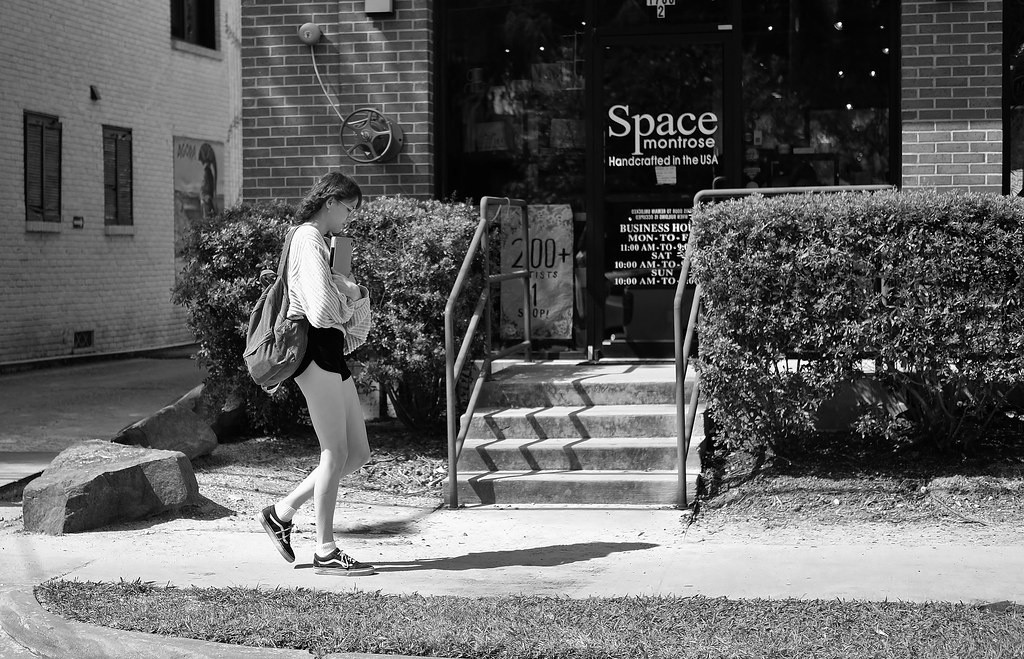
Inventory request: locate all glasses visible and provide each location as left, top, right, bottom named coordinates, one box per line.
left=337, top=200, right=356, bottom=217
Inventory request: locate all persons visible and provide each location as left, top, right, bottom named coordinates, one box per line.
left=257, top=170, right=376, bottom=577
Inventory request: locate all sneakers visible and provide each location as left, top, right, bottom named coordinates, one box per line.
left=313, top=547, right=375, bottom=575
left=258, top=505, right=296, bottom=564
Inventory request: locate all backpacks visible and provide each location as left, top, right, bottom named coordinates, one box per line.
left=243, top=225, right=310, bottom=394
